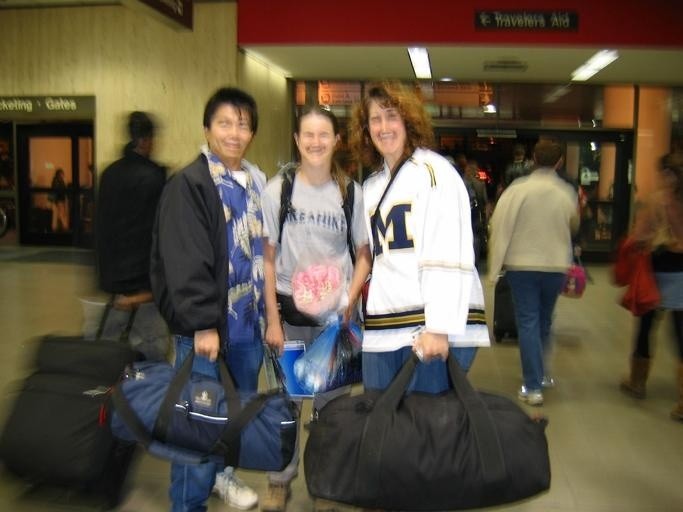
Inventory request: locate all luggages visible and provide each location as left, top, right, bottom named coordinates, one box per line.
left=1, top=320, right=154, bottom=499
left=491, top=278, right=519, bottom=345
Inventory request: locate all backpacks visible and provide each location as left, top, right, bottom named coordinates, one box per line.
left=563, top=256, right=588, bottom=301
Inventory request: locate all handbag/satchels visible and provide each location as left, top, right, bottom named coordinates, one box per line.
left=105, top=335, right=300, bottom=473
left=299, top=347, right=552, bottom=508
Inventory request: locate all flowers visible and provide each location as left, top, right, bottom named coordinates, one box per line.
left=289, top=260, right=347, bottom=319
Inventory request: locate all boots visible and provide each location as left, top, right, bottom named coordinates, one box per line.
left=619, top=350, right=655, bottom=400
left=671, top=362, right=683, bottom=424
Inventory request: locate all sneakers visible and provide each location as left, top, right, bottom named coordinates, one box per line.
left=258, top=478, right=296, bottom=510
left=517, top=375, right=555, bottom=408
left=211, top=469, right=257, bottom=509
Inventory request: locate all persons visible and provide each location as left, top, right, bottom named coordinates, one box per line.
left=442, top=134, right=541, bottom=269
left=488, top=138, right=580, bottom=406
left=620, top=151, right=683, bottom=421
left=357, top=78, right=490, bottom=394
left=49, top=167, right=67, bottom=233
left=147, top=84, right=267, bottom=512
left=96, top=111, right=172, bottom=343
left=262, top=107, right=371, bottom=512
